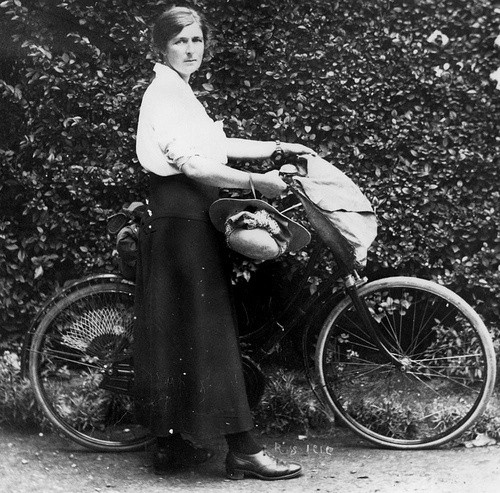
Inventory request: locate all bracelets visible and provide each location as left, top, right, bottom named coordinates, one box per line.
left=130, top=7, right=317, bottom=479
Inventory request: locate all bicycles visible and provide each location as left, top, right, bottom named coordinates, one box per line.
left=21, top=154, right=496, bottom=452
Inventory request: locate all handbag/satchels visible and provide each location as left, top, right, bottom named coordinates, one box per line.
left=286, top=150, right=380, bottom=267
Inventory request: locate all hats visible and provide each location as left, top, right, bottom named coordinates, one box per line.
left=209, top=197, right=312, bottom=260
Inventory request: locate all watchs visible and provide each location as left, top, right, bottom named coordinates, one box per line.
left=269, top=140, right=284, bottom=163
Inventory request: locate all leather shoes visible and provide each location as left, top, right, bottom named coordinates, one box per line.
left=225, top=444, right=302, bottom=480
left=154, top=438, right=215, bottom=475
